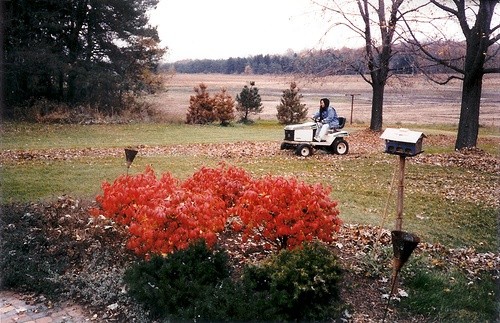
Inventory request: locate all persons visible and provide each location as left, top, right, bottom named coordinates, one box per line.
left=311, top=98, right=339, bottom=142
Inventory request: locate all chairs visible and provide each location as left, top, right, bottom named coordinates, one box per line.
left=329, top=117, right=347, bottom=133
